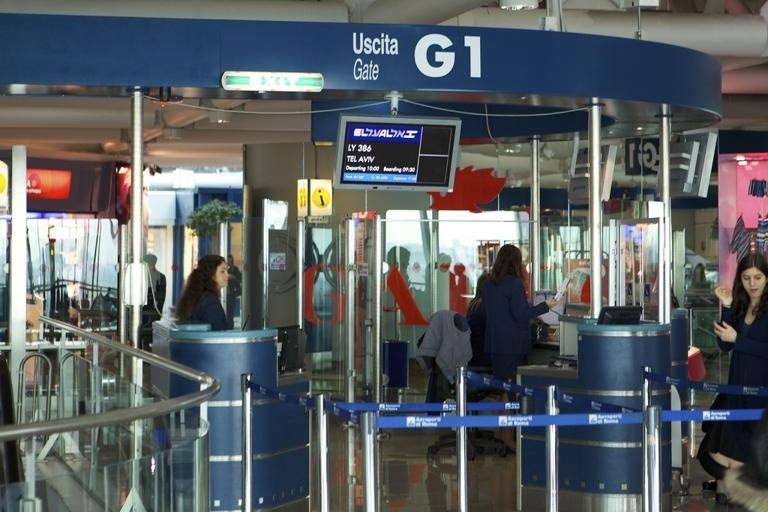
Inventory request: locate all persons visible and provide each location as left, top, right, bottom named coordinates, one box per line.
left=696, top=254, right=768, bottom=512
left=138, top=254, right=166, bottom=397
left=175, top=255, right=229, bottom=330
left=467, top=244, right=557, bottom=454
left=227, top=254, right=242, bottom=330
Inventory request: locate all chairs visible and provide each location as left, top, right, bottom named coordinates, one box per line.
left=427, top=309, right=508, bottom=462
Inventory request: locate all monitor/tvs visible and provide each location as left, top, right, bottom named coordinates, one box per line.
left=596, top=306, right=643, bottom=324
left=655, top=131, right=718, bottom=198
left=333, top=116, right=463, bottom=194
left=567, top=144, right=618, bottom=206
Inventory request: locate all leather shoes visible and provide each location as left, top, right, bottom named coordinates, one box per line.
left=702, top=480, right=727, bottom=506
